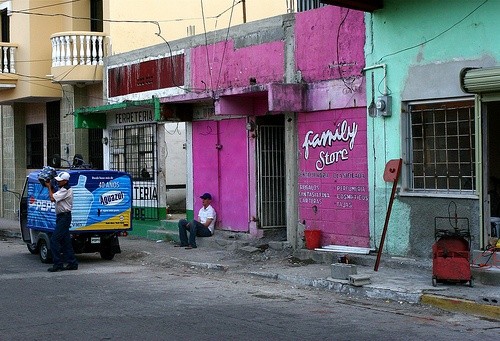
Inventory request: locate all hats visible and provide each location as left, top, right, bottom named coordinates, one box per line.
left=54, top=172, right=70, bottom=182
left=200, top=193, right=212, bottom=200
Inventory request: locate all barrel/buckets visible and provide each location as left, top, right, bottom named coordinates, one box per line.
left=304, top=230, right=322, bottom=250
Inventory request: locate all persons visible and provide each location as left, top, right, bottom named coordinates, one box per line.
left=45, top=172, right=78, bottom=272
left=175, top=193, right=216, bottom=249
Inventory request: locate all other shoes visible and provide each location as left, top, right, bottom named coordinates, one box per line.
left=47, top=263, right=63, bottom=272
left=61, top=263, right=78, bottom=270
left=179, top=242, right=189, bottom=247
left=187, top=243, right=197, bottom=248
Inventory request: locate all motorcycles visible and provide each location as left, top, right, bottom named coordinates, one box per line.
left=3, top=168, right=133, bottom=264
